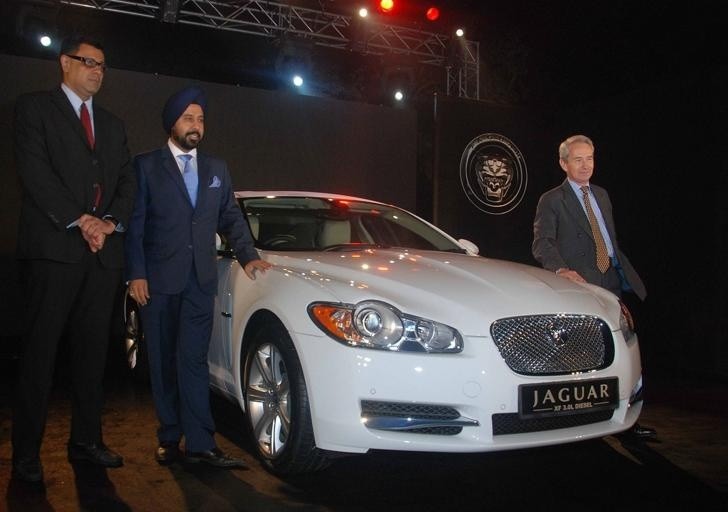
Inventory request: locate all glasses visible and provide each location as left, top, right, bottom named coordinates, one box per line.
left=67, top=55, right=108, bottom=73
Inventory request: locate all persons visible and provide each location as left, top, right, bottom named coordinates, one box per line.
left=120, top=84, right=276, bottom=470
left=0, top=34, right=138, bottom=485
left=530, top=134, right=660, bottom=439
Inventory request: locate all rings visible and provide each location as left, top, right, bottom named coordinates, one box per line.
left=129, top=290, right=135, bottom=297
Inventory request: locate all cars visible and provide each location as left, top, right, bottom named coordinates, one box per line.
left=119, top=191, right=647, bottom=478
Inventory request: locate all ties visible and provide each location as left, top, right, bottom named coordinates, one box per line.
left=580, top=186, right=610, bottom=273
left=179, top=155, right=198, bottom=208
left=80, top=103, right=101, bottom=207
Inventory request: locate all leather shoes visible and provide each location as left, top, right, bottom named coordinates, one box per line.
left=185, top=447, right=240, bottom=466
left=13, top=447, right=42, bottom=482
left=623, top=424, right=657, bottom=438
left=68, top=444, right=123, bottom=467
left=155, top=442, right=179, bottom=461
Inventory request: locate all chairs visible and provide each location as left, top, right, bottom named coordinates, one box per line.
left=316, top=217, right=350, bottom=251
